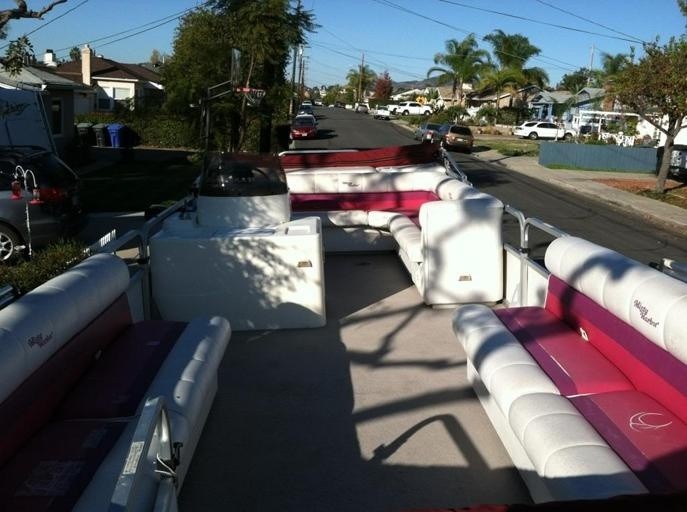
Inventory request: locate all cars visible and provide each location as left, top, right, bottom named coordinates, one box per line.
left=292, top=97, right=325, bottom=139
left=513, top=121, right=577, bottom=141
left=328, top=100, right=435, bottom=120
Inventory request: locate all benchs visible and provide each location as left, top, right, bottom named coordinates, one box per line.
left=146, top=215, right=328, bottom=333
left=251, top=163, right=507, bottom=312
left=0, top=249, right=236, bottom=508
left=450, top=231, right=686, bottom=509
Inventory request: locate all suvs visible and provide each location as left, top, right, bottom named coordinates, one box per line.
left=414, top=122, right=443, bottom=144
left=432, top=125, right=474, bottom=153
left=0, top=143, right=89, bottom=269
